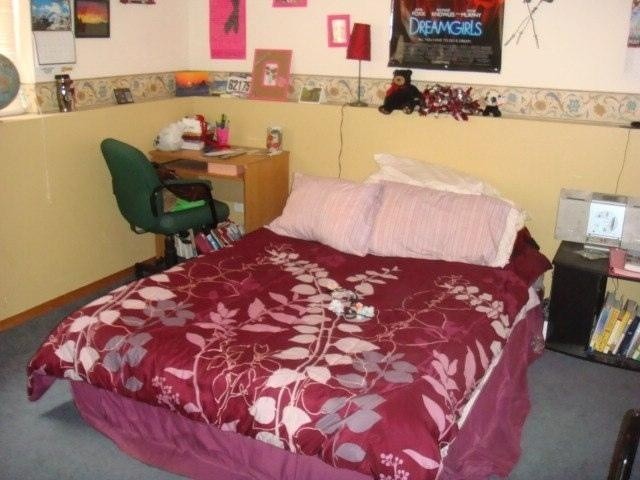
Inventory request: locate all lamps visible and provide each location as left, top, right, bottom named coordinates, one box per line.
left=346, top=22, right=370, bottom=108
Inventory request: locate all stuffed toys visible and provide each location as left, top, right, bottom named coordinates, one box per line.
left=480, top=89, right=503, bottom=116
left=377, top=69, right=422, bottom=115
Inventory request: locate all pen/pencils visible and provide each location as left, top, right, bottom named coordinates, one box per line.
left=215, top=114, right=230, bottom=129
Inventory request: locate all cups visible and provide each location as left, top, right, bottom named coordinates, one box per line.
left=55, top=75, right=73, bottom=113
left=216, top=127, right=229, bottom=145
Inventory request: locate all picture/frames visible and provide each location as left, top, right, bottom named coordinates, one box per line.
left=326, top=14, right=352, bottom=48
left=248, top=48, right=292, bottom=102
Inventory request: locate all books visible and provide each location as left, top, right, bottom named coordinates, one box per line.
left=174, top=220, right=243, bottom=260
left=607, top=248, right=640, bottom=282
left=588, top=291, right=640, bottom=363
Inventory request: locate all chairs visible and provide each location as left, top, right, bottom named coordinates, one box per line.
left=100, top=138, right=230, bottom=276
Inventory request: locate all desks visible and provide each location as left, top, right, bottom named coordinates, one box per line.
left=147, top=145, right=289, bottom=258
left=544, top=240, right=640, bottom=374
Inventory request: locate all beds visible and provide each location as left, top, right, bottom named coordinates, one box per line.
left=26, top=228, right=553, bottom=479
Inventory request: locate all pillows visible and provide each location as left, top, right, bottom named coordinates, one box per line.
left=370, top=180, right=528, bottom=269
left=263, top=172, right=381, bottom=262
left=364, top=152, right=512, bottom=202
